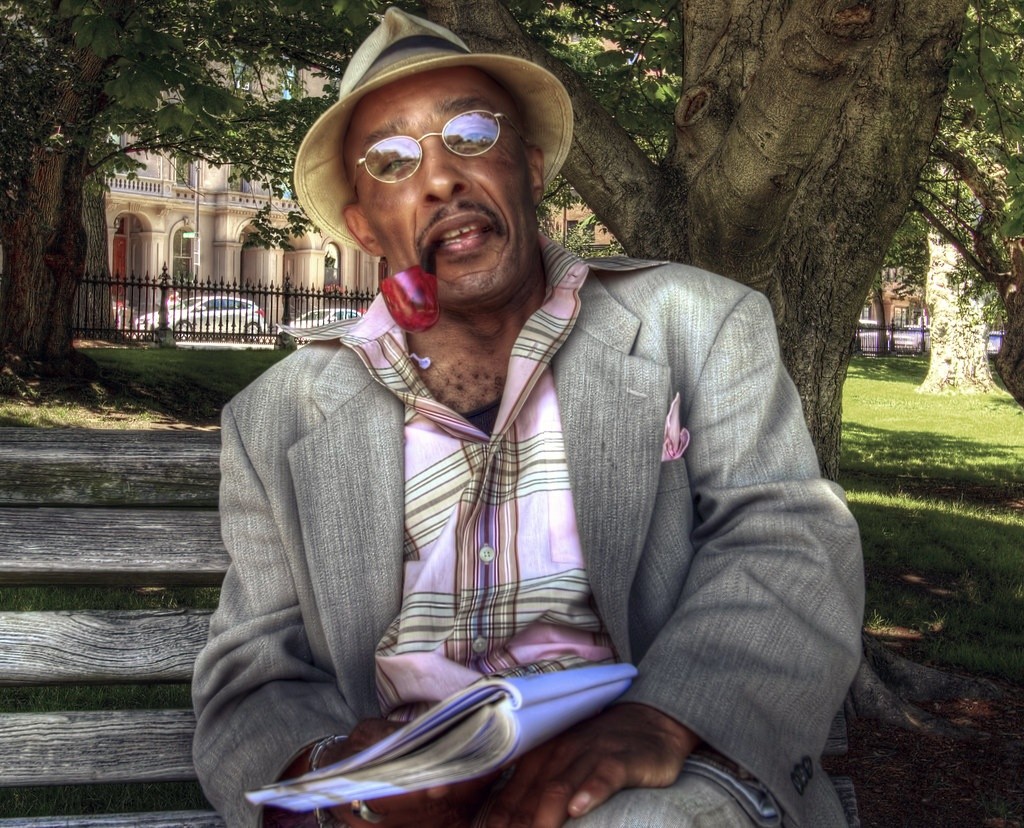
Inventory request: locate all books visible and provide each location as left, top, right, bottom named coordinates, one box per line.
left=243, top=660, right=638, bottom=814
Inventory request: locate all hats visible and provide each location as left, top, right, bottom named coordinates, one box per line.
left=294, top=6, right=574, bottom=249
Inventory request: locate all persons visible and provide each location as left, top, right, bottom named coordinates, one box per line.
left=192, top=5, right=867, bottom=828
left=168, top=291, right=182, bottom=302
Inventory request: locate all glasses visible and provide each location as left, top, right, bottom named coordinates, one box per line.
left=353, top=109, right=529, bottom=203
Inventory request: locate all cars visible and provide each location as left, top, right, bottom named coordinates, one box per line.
left=987, top=330, right=1004, bottom=355
left=890, top=324, right=932, bottom=354
left=134, top=295, right=271, bottom=335
left=276, top=308, right=363, bottom=336
left=853, top=320, right=891, bottom=354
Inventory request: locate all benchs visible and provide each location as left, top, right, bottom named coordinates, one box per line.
left=0, top=429, right=857, bottom=828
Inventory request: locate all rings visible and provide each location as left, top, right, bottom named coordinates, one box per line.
left=352, top=800, right=388, bottom=824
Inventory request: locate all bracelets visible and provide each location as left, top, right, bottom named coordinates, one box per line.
left=308, top=734, right=350, bottom=828
